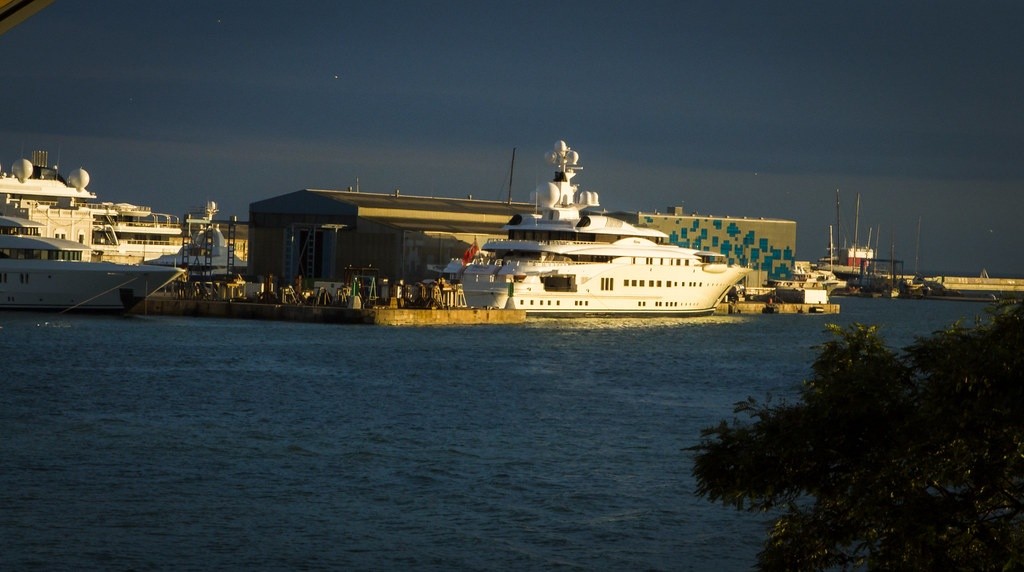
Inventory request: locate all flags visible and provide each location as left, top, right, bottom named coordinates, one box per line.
left=463, top=242, right=479, bottom=262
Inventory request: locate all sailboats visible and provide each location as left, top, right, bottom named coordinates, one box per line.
left=882, top=240, right=899, bottom=299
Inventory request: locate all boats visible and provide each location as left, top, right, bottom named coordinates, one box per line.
left=143, top=199, right=249, bottom=279
left=795, top=226, right=847, bottom=290
left=2, top=214, right=187, bottom=316
left=0, top=149, right=187, bottom=265
left=906, top=278, right=932, bottom=296
left=421, top=139, right=755, bottom=319
